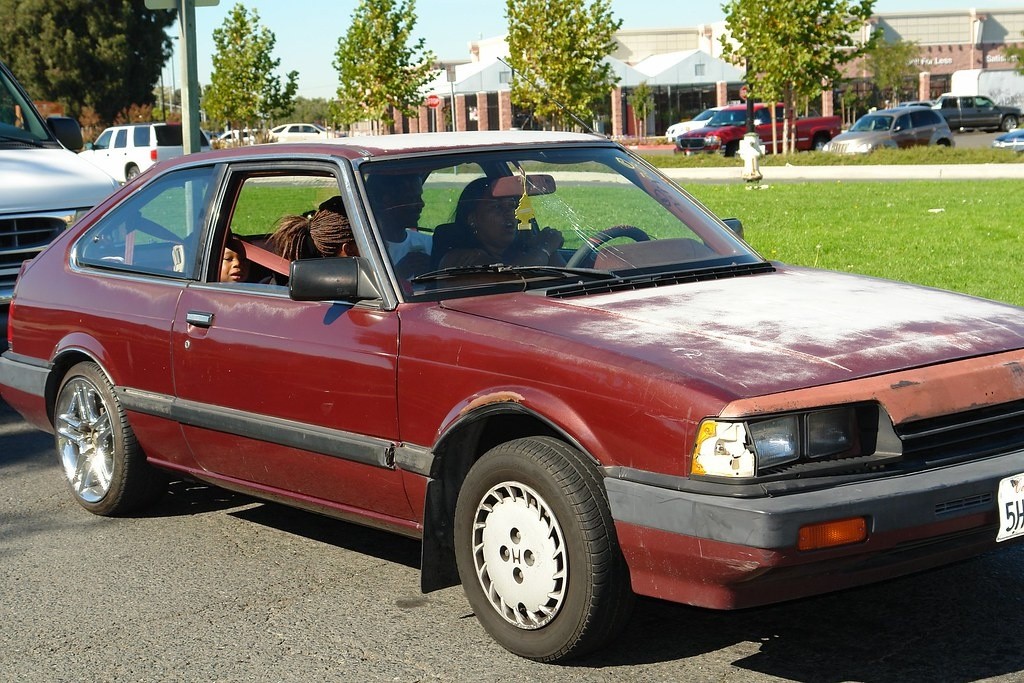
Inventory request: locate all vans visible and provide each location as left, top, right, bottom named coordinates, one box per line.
left=0, top=52, right=123, bottom=345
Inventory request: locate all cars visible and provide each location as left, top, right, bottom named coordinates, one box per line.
left=990, top=126, right=1024, bottom=154
left=202, top=121, right=345, bottom=147
left=0, top=130, right=1024, bottom=663
left=664, top=104, right=733, bottom=145
left=821, top=106, right=956, bottom=157
left=899, top=100, right=934, bottom=108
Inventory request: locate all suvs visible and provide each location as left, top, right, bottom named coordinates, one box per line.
left=76, top=119, right=216, bottom=189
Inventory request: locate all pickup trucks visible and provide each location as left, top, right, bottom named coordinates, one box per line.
left=672, top=101, right=843, bottom=158
left=927, top=92, right=1021, bottom=133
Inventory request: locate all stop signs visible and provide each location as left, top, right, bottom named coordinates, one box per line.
left=426, top=94, right=440, bottom=109
left=738, top=86, right=748, bottom=101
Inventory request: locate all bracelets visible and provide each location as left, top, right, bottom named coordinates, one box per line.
left=541, top=246, right=551, bottom=260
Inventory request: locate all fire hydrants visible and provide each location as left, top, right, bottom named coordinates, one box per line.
left=735, top=131, right=770, bottom=184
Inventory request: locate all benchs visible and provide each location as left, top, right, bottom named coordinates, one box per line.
left=103, top=234, right=273, bottom=271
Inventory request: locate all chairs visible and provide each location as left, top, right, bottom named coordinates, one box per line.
left=430, top=223, right=532, bottom=271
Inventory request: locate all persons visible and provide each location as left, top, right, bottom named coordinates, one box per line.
left=219, top=167, right=567, bottom=292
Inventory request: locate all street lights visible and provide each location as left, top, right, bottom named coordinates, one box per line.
left=166, top=35, right=181, bottom=112
